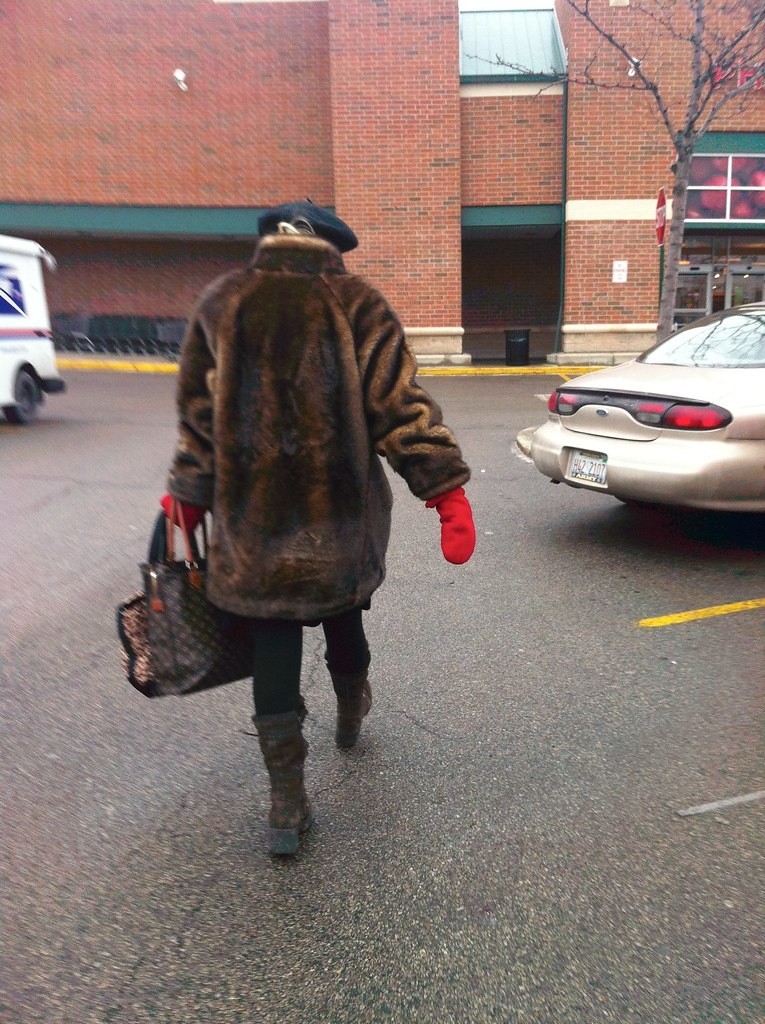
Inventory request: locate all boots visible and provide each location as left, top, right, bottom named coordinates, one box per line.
left=323, top=650, right=373, bottom=748
left=240, top=695, right=312, bottom=855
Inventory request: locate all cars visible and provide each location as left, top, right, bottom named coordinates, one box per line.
left=530, top=302, right=764, bottom=519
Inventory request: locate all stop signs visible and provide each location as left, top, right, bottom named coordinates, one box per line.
left=655, top=185, right=668, bottom=246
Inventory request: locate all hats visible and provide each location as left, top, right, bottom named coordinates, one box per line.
left=257, top=194, right=358, bottom=254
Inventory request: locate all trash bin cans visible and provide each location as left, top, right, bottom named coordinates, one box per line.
left=504, top=328, right=532, bottom=366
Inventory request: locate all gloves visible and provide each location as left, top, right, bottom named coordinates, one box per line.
left=425, top=486, right=476, bottom=565
left=158, top=494, right=207, bottom=532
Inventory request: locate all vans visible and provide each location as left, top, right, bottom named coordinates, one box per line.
left=0, top=233, right=65, bottom=425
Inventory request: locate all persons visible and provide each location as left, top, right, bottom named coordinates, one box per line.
left=155, top=200, right=476, bottom=857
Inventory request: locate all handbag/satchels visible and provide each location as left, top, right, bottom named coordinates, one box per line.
left=116, top=496, right=252, bottom=698
